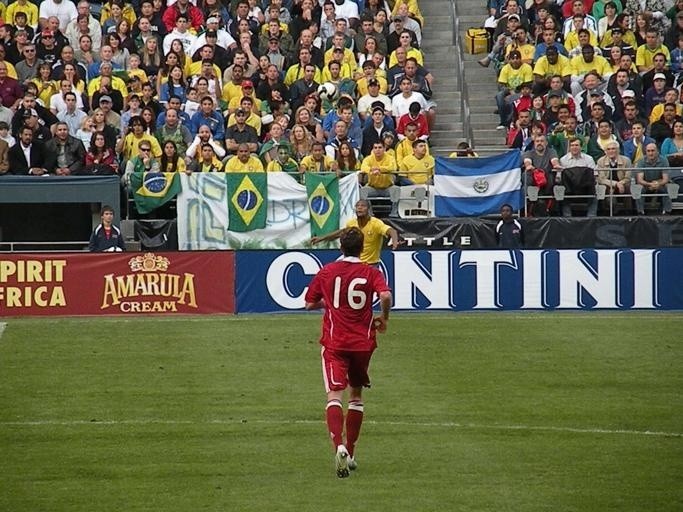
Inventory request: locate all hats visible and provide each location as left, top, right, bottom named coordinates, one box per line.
left=533, top=168, right=546, bottom=185
left=368, top=78, right=384, bottom=115
left=128, top=75, right=140, bottom=102
left=393, top=14, right=403, bottom=21
left=653, top=73, right=666, bottom=81
left=206, top=28, right=217, bottom=37
left=269, top=36, right=278, bottom=40
left=99, top=95, right=112, bottom=102
left=548, top=88, right=636, bottom=99
left=508, top=13, right=521, bottom=58
left=235, top=80, right=253, bottom=115
left=22, top=108, right=38, bottom=117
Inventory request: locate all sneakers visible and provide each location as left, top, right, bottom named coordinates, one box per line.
left=496, top=124, right=506, bottom=130
left=336, top=444, right=350, bottom=477
left=493, top=110, right=499, bottom=114
left=477, top=57, right=491, bottom=67
left=348, top=454, right=357, bottom=470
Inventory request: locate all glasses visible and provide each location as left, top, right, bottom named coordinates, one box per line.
left=270, top=42, right=278, bottom=44
left=26, top=50, right=34, bottom=52
left=41, top=35, right=52, bottom=39
left=140, top=148, right=151, bottom=151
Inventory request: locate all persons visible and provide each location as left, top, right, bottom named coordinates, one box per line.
left=304, top=226, right=393, bottom=480
left=310, top=199, right=407, bottom=270
left=494, top=205, right=527, bottom=250
left=87, top=207, right=127, bottom=253
left=477, top=0, right=683, bottom=217
left=0, top=0, right=437, bottom=232
left=449, top=143, right=476, bottom=157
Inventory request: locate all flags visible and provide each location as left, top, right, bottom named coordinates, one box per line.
left=433, top=147, right=524, bottom=216
